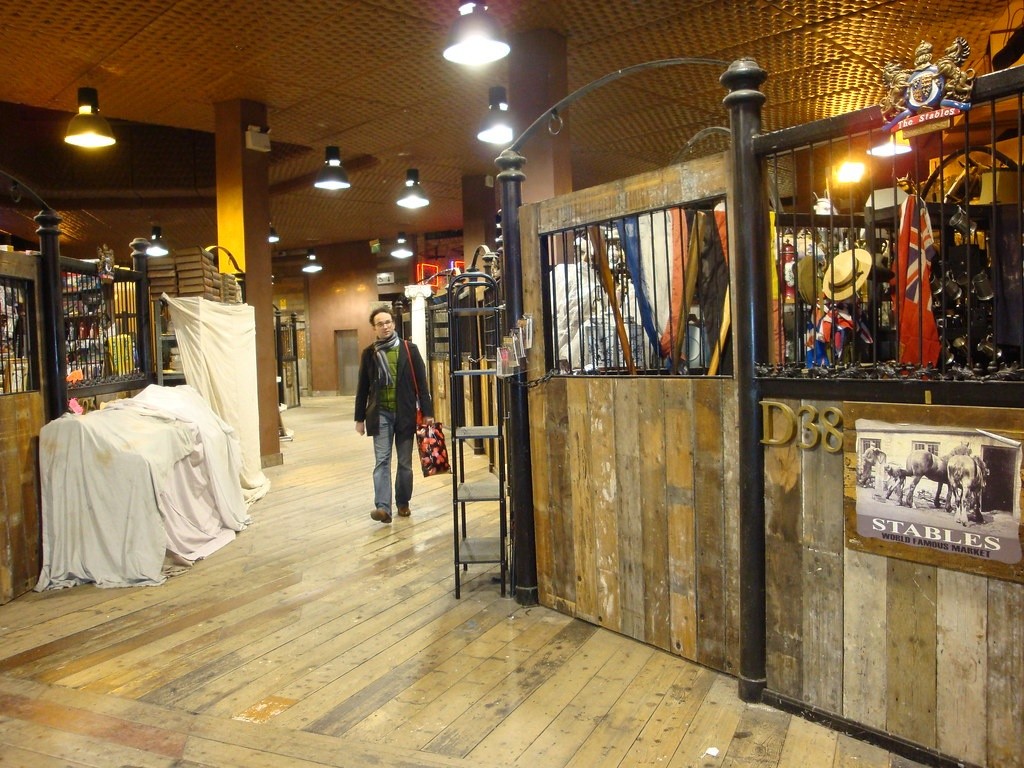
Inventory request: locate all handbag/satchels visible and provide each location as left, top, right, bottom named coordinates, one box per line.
left=416, top=423, right=453, bottom=477
left=952, top=230, right=987, bottom=275
left=416, top=409, right=423, bottom=427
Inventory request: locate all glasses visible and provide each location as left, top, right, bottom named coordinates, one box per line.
left=374, top=320, right=393, bottom=327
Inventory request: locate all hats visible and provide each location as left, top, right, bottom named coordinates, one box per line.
left=823, top=248, right=872, bottom=300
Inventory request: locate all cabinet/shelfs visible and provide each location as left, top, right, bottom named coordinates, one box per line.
left=447, top=273, right=508, bottom=599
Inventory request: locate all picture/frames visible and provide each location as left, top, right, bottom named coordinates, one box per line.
left=843, top=400, right=1024, bottom=585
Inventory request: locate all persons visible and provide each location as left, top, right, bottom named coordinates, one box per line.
left=859, top=443, right=886, bottom=488
left=884, top=464, right=904, bottom=506
left=354, top=308, right=434, bottom=523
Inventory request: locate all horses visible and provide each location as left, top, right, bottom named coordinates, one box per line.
left=897, top=439, right=988, bottom=527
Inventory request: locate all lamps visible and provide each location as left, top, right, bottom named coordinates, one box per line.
left=63, top=86, right=117, bottom=148
left=867, top=127, right=913, bottom=158
left=268, top=220, right=280, bottom=243
left=391, top=231, right=414, bottom=260
left=477, top=85, right=513, bottom=146
left=442, top=0, right=510, bottom=67
left=313, top=146, right=351, bottom=191
left=396, top=169, right=430, bottom=209
left=146, top=225, right=168, bottom=257
left=302, top=249, right=322, bottom=273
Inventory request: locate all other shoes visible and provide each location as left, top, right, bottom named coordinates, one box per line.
left=371, top=509, right=392, bottom=523
left=398, top=506, right=411, bottom=517
